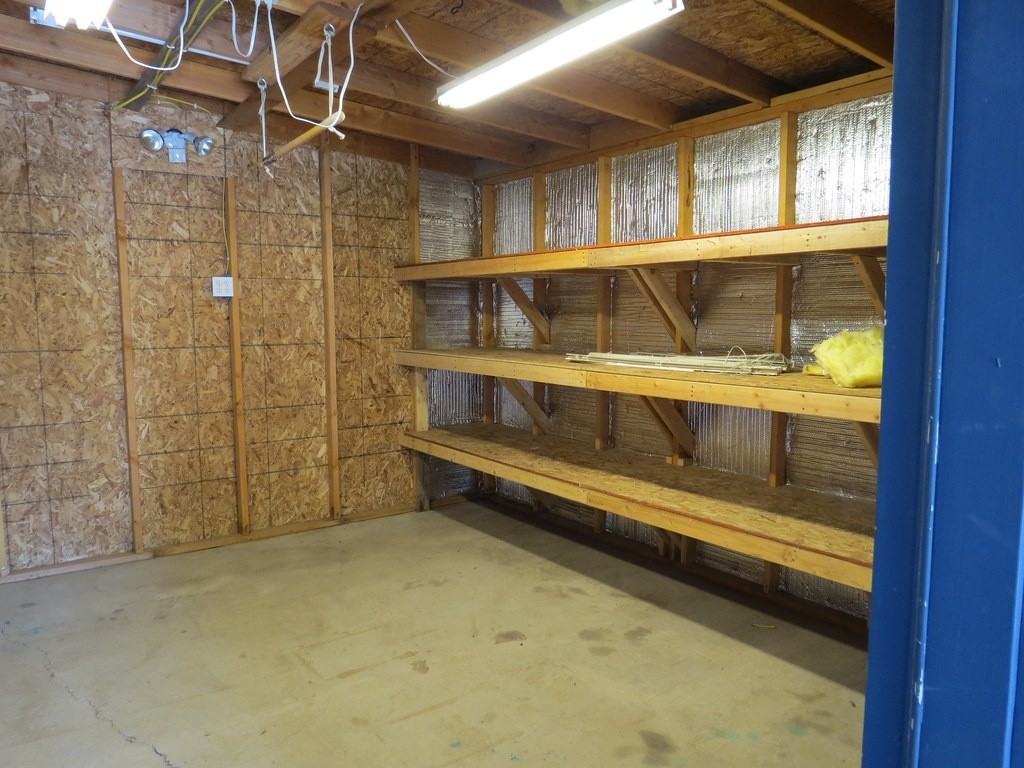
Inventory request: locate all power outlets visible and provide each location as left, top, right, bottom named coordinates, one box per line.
left=211, top=276, right=234, bottom=298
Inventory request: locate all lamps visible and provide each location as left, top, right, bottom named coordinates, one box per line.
left=430, top=0, right=686, bottom=111
left=140, top=128, right=216, bottom=164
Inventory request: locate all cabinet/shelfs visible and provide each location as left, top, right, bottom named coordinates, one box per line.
left=390, top=213, right=887, bottom=599
